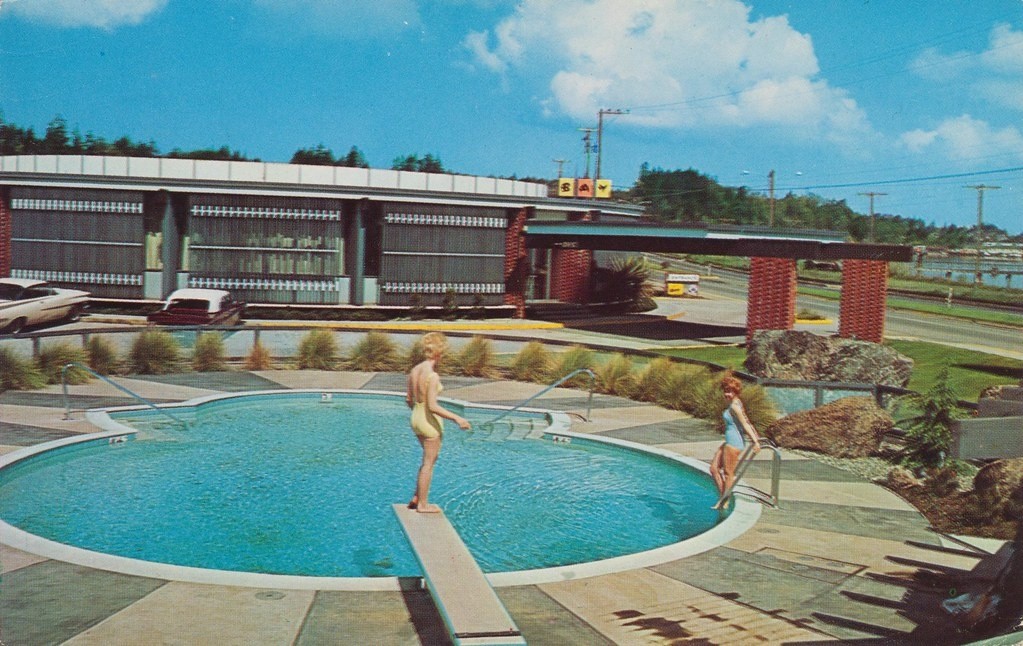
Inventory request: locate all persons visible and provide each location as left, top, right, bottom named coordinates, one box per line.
left=709, top=376, right=761, bottom=509
left=406, top=331, right=471, bottom=513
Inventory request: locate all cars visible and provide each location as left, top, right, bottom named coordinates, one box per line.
left=146, top=288, right=243, bottom=329
left=1, top=277, right=94, bottom=335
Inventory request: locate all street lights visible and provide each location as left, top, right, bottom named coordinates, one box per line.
left=741, top=167, right=804, bottom=230
